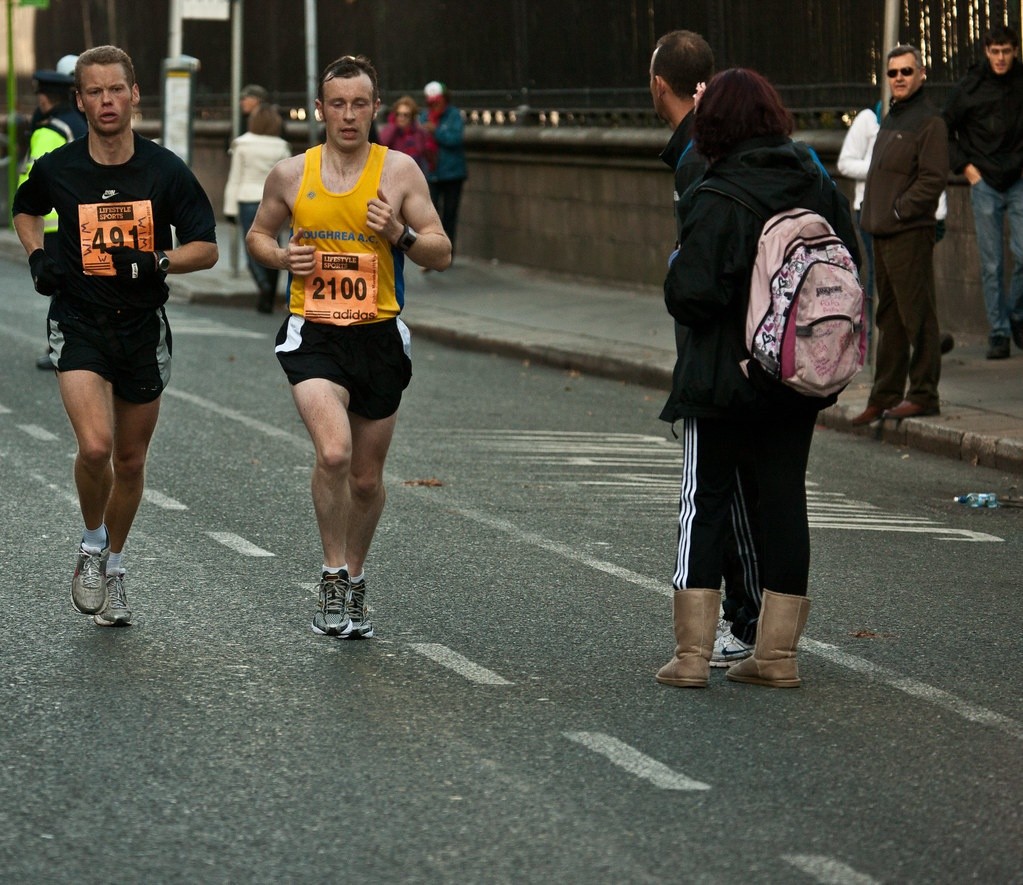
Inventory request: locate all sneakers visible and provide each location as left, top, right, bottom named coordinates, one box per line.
left=708, top=616, right=733, bottom=639
left=93, top=568, right=133, bottom=627
left=337, top=578, right=374, bottom=639
left=69, top=525, right=111, bottom=615
left=708, top=635, right=758, bottom=668
left=312, top=569, right=353, bottom=635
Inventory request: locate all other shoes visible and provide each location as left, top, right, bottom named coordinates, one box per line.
left=36, top=353, right=54, bottom=373
left=985, top=331, right=1011, bottom=358
left=940, top=334, right=954, bottom=354
left=1010, top=313, right=1023, bottom=350
left=253, top=279, right=275, bottom=312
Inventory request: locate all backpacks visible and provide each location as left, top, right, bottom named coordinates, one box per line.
left=691, top=174, right=867, bottom=396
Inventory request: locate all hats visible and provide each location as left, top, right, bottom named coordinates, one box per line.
left=424, top=81, right=445, bottom=99
left=57, top=54, right=80, bottom=76
left=31, top=69, right=76, bottom=100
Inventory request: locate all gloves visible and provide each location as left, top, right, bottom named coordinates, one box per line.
left=26, top=249, right=58, bottom=298
left=387, top=113, right=398, bottom=128
left=105, top=245, right=159, bottom=284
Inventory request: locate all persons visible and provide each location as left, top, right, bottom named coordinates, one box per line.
left=15, top=68, right=467, bottom=371
left=836, top=94, right=953, bottom=355
left=10, top=46, right=219, bottom=628
left=947, top=25, right=1022, bottom=358
left=851, top=45, right=949, bottom=426
left=649, top=26, right=765, bottom=669
left=652, top=67, right=862, bottom=688
left=245, top=58, right=452, bottom=639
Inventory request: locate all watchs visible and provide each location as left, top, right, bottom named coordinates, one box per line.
left=394, top=221, right=418, bottom=252
left=154, top=249, right=170, bottom=276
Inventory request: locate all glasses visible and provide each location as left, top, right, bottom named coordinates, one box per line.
left=395, top=112, right=415, bottom=119
left=886, top=67, right=922, bottom=79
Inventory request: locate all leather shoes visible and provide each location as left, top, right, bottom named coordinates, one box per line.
left=851, top=404, right=888, bottom=426
left=882, top=399, right=941, bottom=418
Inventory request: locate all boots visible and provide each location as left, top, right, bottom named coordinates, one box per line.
left=655, top=588, right=721, bottom=686
left=723, top=586, right=813, bottom=687
